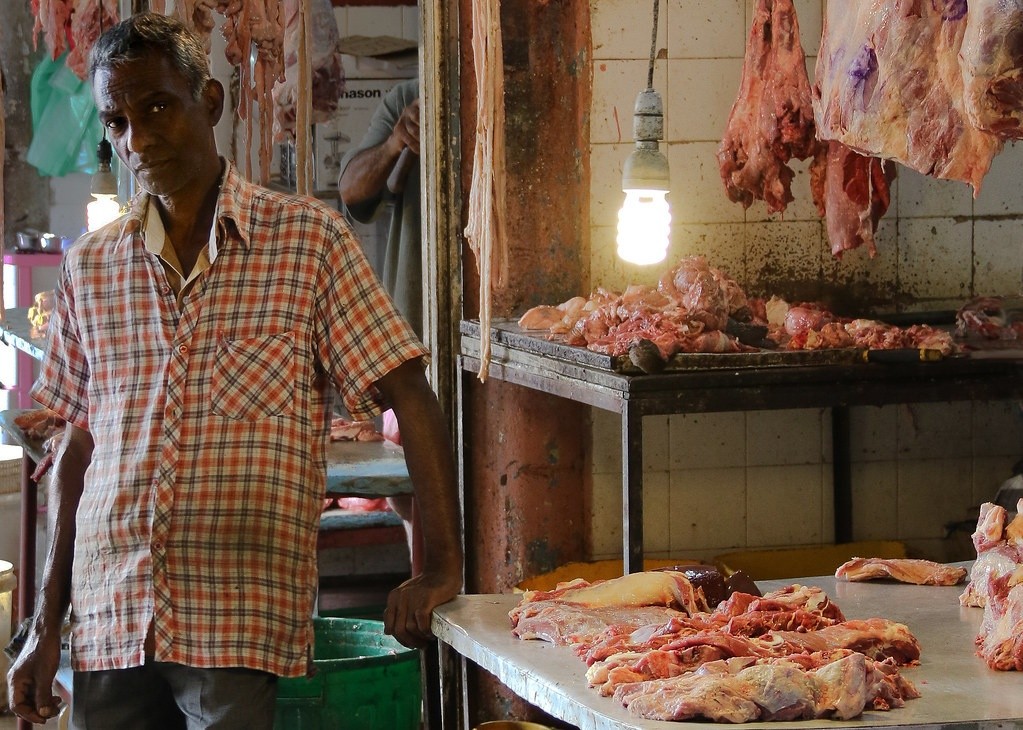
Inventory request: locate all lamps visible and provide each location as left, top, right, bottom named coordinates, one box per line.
left=616, top=0, right=672, bottom=267
left=86, top=126, right=120, bottom=231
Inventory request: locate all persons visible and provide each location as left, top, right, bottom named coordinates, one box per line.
left=4, top=12, right=465, bottom=730
left=338, top=75, right=424, bottom=348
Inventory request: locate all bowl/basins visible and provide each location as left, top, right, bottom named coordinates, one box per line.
left=40, top=237, right=62, bottom=252
left=16, top=233, right=39, bottom=250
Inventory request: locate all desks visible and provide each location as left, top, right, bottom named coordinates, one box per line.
left=420, top=561, right=1023, bottom=730
left=451, top=315, right=1023, bottom=730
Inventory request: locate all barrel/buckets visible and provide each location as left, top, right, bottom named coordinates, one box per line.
left=274, top=615, right=419, bottom=730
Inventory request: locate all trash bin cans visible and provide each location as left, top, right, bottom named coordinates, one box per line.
left=274, top=616, right=424, bottom=730
